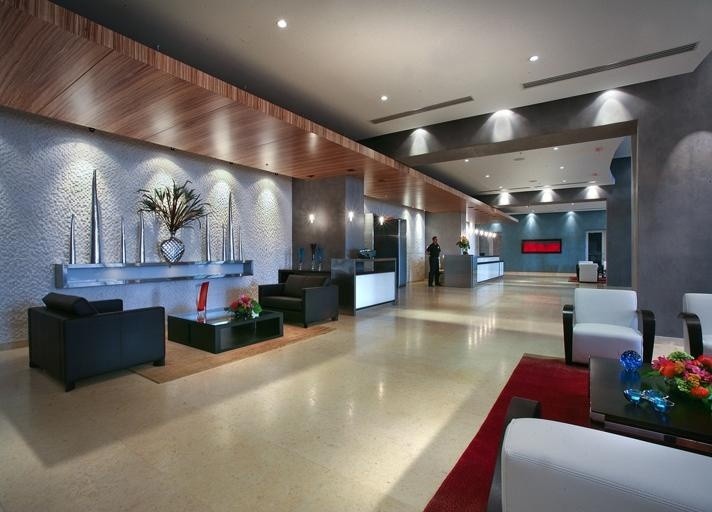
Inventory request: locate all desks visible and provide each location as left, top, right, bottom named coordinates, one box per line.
left=329, top=256, right=400, bottom=317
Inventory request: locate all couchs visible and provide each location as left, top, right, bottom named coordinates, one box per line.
left=255, top=271, right=342, bottom=333
left=482, top=394, right=710, bottom=511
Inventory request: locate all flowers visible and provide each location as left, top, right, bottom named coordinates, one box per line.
left=454, top=236, right=471, bottom=250
left=642, top=348, right=712, bottom=404
left=222, top=293, right=263, bottom=320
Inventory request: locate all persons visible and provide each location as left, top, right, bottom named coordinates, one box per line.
left=426, top=236, right=443, bottom=287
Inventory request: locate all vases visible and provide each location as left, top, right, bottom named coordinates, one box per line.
left=234, top=307, right=253, bottom=320
left=461, top=247, right=468, bottom=255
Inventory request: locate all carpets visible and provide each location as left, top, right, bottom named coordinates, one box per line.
left=418, top=351, right=594, bottom=512
left=130, top=322, right=341, bottom=386
left=567, top=276, right=607, bottom=284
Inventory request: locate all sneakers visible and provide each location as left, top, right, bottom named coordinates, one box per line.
left=435, top=281, right=441, bottom=287
left=428, top=281, right=434, bottom=287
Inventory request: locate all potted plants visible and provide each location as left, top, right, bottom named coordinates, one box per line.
left=131, top=172, right=213, bottom=266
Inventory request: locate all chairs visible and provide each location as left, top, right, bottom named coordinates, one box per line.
left=675, top=289, right=712, bottom=361
left=575, top=260, right=599, bottom=284
left=27, top=289, right=171, bottom=396
left=560, top=286, right=657, bottom=368
